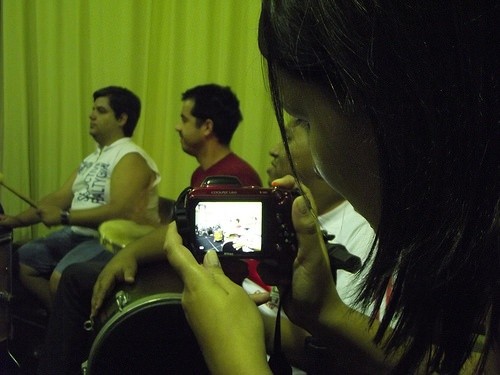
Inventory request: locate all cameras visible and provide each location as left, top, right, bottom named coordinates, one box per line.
left=174, top=176, right=300, bottom=260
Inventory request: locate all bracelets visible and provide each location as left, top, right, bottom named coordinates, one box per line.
left=60, top=207, right=70, bottom=225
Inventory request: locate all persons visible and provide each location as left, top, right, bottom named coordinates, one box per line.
left=90, top=119, right=402, bottom=375
left=38, top=82, right=262, bottom=375
left=160, top=1, right=499, bottom=374
left=0, top=85, right=162, bottom=375
